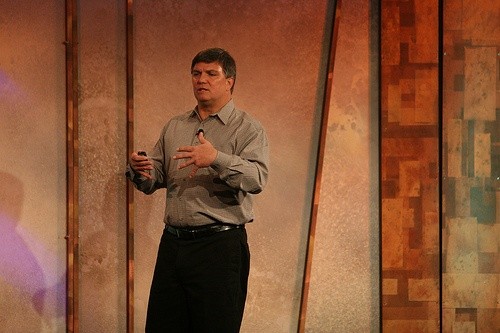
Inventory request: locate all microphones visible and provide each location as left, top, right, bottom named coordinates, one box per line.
left=195, top=129, right=204, bottom=136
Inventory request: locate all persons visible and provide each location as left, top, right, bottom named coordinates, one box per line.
left=126, top=47, right=269, bottom=332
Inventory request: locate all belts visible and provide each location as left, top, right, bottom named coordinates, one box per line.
left=165, top=223, right=242, bottom=236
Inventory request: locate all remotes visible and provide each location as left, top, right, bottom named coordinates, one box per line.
left=138, top=151, right=148, bottom=160
